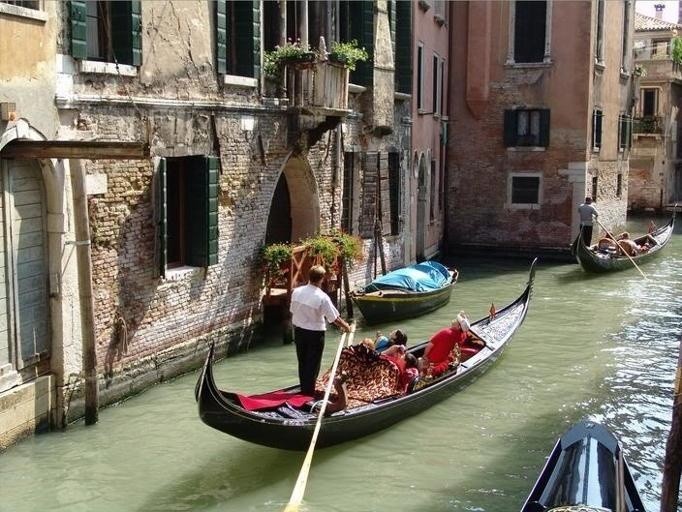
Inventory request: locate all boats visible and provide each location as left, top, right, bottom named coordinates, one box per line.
left=189, top=255, right=546, bottom=455
left=569, top=214, right=681, bottom=280
left=518, top=418, right=649, bottom=511
left=344, top=259, right=460, bottom=322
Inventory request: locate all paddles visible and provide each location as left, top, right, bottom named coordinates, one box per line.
left=289, top=330, right=347, bottom=505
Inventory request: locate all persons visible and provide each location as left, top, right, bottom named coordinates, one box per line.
left=578, top=196, right=598, bottom=247
left=363, top=311, right=471, bottom=394
left=606, top=232, right=641, bottom=255
left=289, top=265, right=349, bottom=397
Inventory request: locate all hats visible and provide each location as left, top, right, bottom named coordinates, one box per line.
left=457, top=310, right=470, bottom=332
